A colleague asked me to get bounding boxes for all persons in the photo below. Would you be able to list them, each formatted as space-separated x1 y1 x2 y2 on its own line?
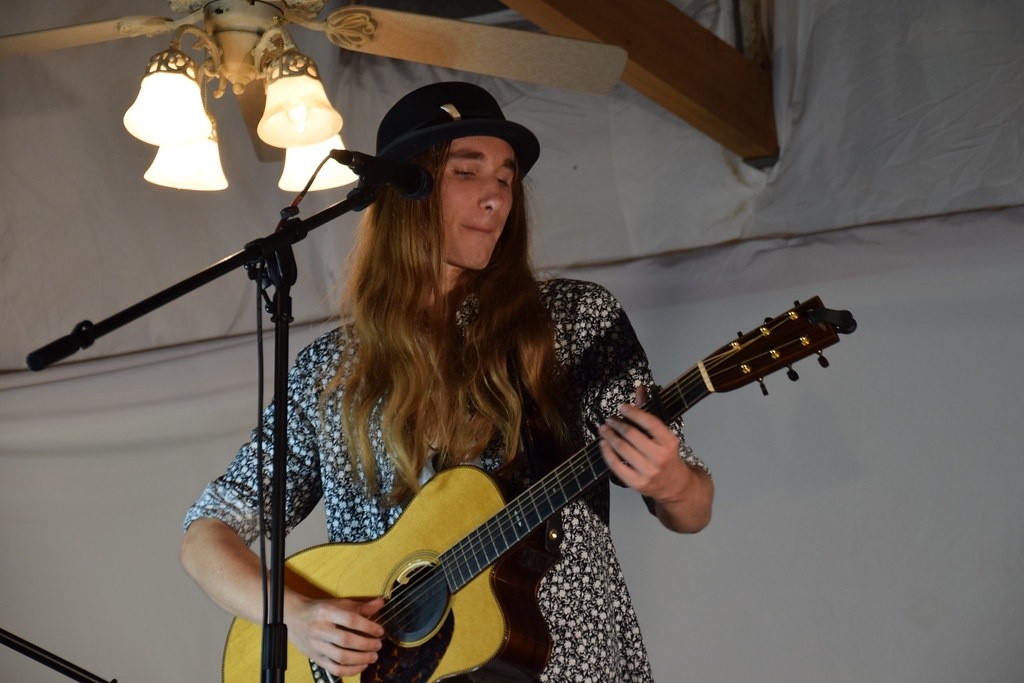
181 81 715 683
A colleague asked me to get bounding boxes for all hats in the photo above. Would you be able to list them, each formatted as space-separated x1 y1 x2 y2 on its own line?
376 82 540 179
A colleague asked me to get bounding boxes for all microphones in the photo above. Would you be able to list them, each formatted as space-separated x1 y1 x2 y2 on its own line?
330 150 434 200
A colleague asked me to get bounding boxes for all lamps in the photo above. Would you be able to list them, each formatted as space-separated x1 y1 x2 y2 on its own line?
123 23 359 193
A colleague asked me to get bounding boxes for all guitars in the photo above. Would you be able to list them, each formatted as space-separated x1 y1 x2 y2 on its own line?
219 294 860 683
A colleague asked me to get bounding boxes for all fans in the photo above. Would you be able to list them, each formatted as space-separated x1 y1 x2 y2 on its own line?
0 0 627 162
